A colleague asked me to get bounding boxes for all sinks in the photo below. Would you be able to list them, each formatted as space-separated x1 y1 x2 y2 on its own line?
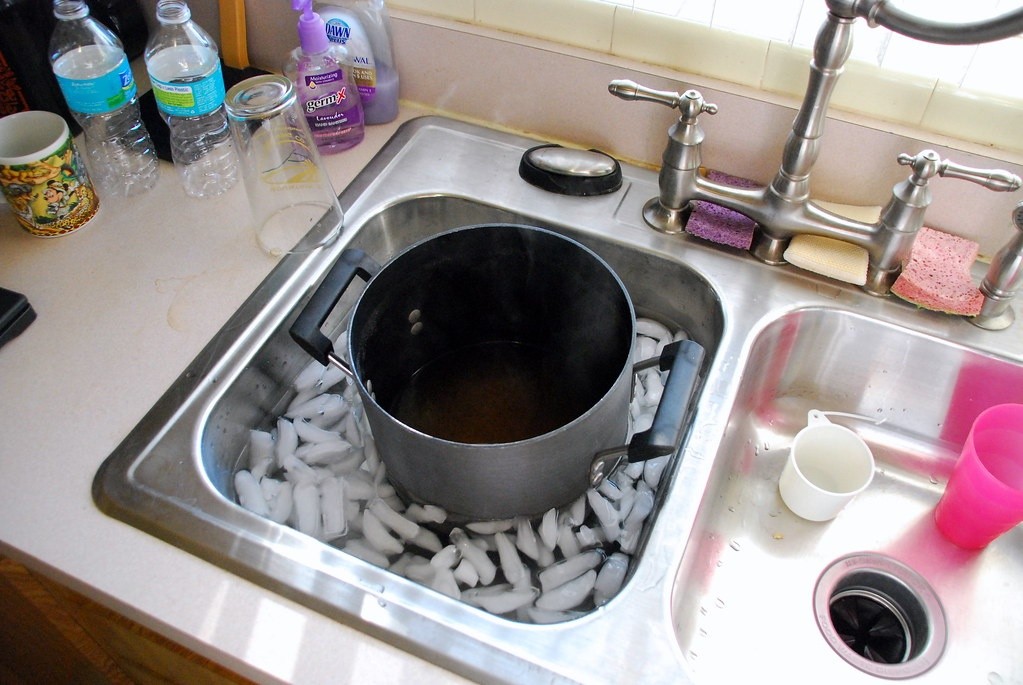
91 115 729 685
664 302 1023 685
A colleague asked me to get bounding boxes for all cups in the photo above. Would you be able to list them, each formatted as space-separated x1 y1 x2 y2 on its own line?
778 409 875 522
933 402 1022 551
224 74 344 254
0 110 101 239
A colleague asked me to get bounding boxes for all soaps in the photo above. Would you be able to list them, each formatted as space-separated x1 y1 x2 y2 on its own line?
528 147 617 177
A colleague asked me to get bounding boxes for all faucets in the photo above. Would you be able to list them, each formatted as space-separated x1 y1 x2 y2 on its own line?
604 0 1023 298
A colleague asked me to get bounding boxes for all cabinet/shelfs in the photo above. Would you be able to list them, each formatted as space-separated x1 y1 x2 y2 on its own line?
0 556 257 685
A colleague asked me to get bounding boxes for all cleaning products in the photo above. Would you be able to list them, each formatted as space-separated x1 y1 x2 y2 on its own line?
280 1 402 158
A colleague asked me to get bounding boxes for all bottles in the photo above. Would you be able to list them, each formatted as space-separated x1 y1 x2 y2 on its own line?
48 0 159 198
312 0 400 125
144 0 240 200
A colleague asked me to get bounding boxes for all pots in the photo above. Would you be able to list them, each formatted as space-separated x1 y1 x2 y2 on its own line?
287 222 706 534
